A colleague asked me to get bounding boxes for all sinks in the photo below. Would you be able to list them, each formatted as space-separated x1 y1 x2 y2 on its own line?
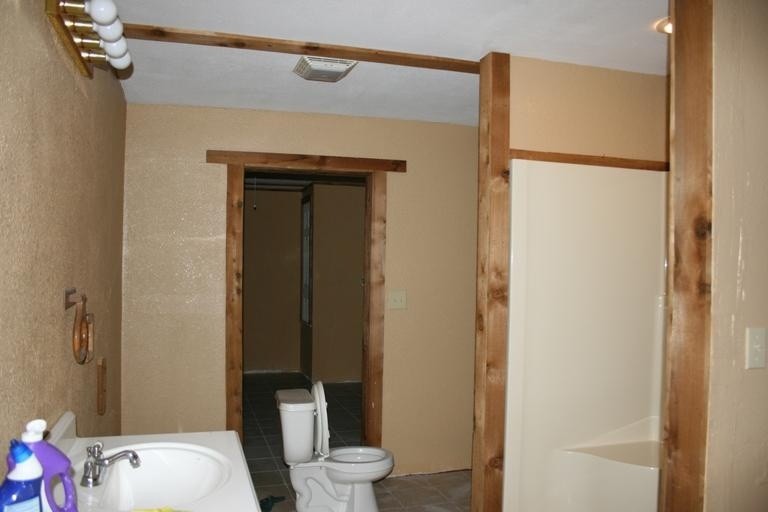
98 441 233 512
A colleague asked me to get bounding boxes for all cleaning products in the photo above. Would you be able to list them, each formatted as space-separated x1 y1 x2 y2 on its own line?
0 419 76 512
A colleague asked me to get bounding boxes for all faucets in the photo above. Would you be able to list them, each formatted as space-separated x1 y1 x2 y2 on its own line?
93 449 142 478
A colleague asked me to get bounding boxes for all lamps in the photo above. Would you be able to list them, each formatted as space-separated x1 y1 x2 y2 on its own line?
47 0 135 77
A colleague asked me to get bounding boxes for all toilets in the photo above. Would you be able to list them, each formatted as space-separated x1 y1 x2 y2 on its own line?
276 381 394 512
